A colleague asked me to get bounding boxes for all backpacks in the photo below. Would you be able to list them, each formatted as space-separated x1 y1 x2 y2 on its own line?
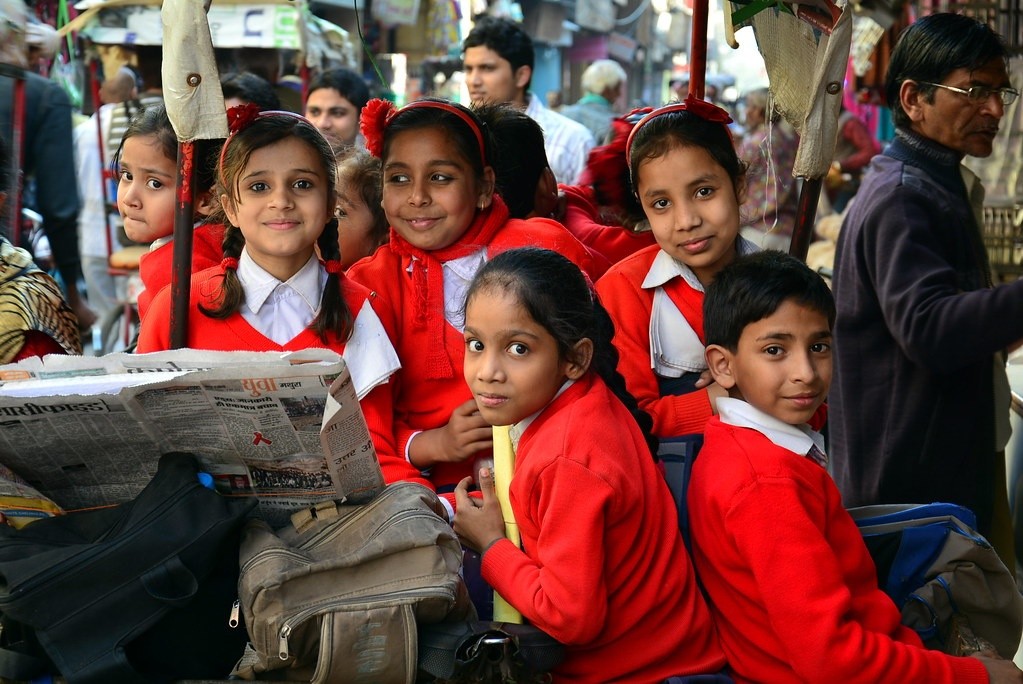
0 450 257 684
229 478 477 684
848 502 1023 659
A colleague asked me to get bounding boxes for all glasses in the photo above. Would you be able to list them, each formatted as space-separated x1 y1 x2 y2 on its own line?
920 79 1020 106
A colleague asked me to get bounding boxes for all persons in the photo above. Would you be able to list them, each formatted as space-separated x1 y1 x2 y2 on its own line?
673 74 873 253
562 106 654 265
0 139 83 363
109 108 231 317
688 251 1023 684
475 100 558 221
334 151 392 266
594 95 830 518
73 70 167 354
462 19 630 189
0 0 99 329
432 245 730 684
344 98 592 616
221 68 368 160
828 13 1023 572
138 111 434 490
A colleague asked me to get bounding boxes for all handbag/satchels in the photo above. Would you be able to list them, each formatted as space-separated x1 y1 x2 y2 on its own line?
416 619 559 684
